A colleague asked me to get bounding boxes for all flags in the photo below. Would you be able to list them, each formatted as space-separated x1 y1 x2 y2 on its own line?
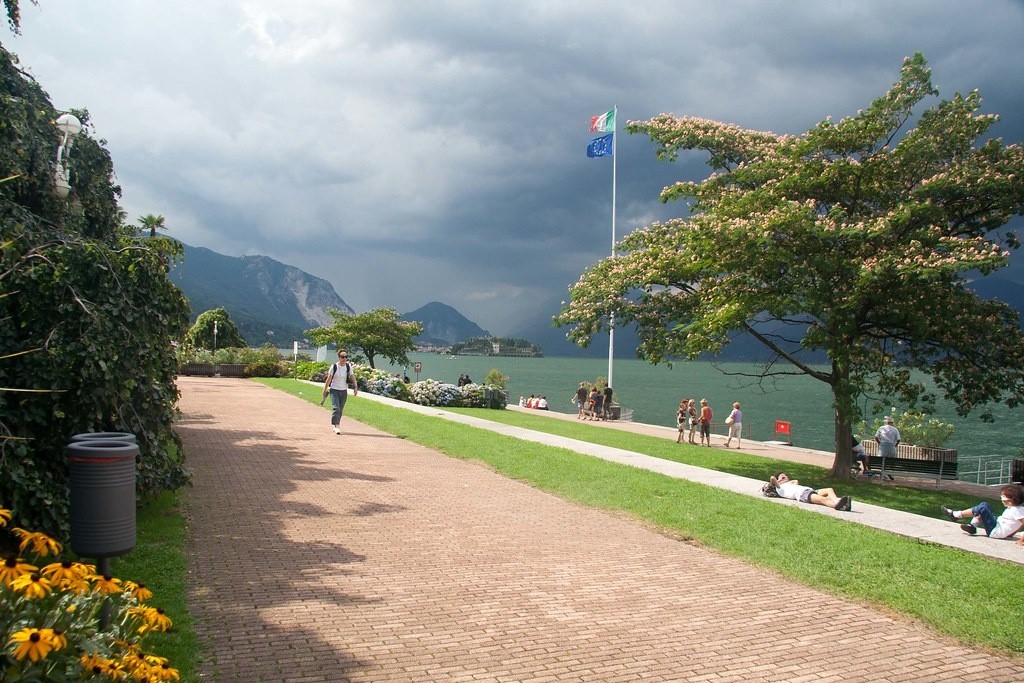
586 132 614 158
588 109 615 132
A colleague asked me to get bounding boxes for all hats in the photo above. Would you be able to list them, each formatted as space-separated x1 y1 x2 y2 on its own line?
886 418 894 422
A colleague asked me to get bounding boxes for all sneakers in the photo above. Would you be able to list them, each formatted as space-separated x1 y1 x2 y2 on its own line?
835 496 848 510
844 496 852 511
334 428 341 435
941 505 958 523
961 523 976 534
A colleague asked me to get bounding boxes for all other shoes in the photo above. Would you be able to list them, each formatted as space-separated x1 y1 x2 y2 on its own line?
888 475 895 480
677 440 740 448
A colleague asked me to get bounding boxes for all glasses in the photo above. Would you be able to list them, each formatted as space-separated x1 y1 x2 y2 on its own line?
338 355 347 358
777 476 789 482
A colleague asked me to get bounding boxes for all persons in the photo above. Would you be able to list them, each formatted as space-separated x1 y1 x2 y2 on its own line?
770 471 851 511
677 398 713 447
458 374 472 387
519 394 548 410
941 484 1024 546
323 349 358 435
405 376 410 383
724 402 743 449
573 382 613 421
851 434 873 476
874 417 901 481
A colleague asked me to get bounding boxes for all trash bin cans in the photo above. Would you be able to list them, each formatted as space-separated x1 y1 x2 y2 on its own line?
610 405 620 420
65 432 138 558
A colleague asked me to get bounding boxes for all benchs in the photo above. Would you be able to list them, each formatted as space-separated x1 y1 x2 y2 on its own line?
853 451 958 489
